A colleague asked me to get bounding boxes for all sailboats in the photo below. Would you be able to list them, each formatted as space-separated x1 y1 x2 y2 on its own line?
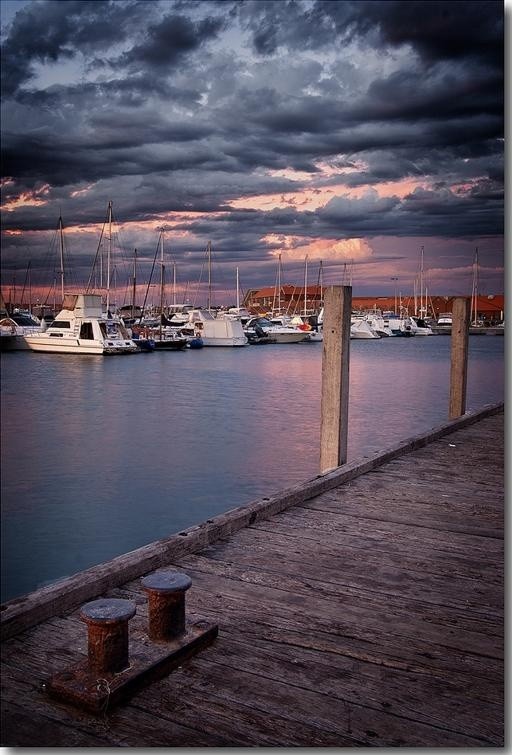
0 200 504 353
23 201 138 356
